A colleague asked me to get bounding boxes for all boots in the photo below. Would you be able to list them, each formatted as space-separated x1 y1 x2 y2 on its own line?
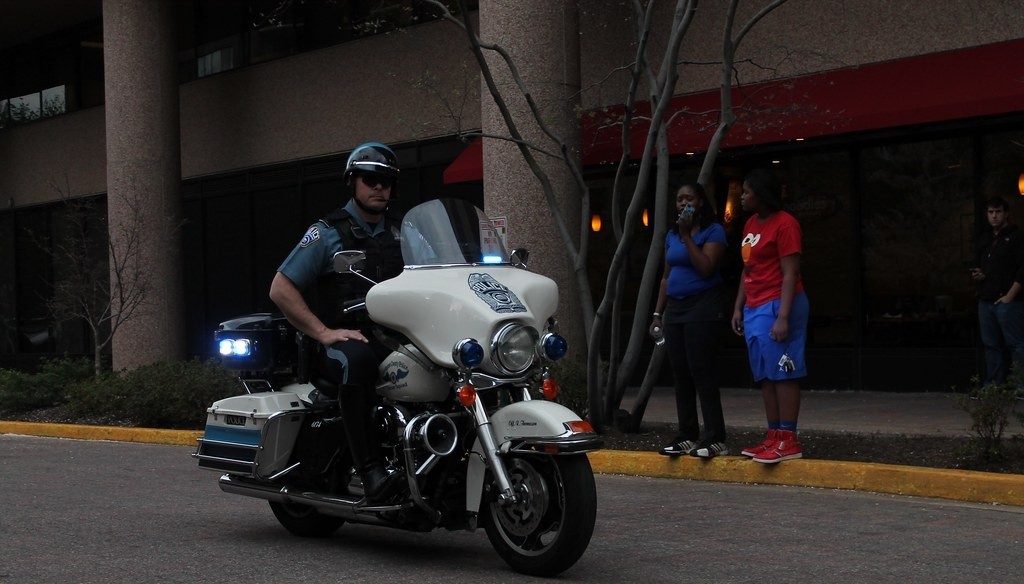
338 383 408 505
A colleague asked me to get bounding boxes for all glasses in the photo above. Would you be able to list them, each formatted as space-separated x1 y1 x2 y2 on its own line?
358 175 394 189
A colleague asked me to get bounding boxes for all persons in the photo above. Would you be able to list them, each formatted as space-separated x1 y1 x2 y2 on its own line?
728 171 810 464
269 144 437 506
646 182 732 457
970 197 1024 400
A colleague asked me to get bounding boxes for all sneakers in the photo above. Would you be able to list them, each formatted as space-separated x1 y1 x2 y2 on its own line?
657 440 701 455
741 429 803 462
689 440 729 458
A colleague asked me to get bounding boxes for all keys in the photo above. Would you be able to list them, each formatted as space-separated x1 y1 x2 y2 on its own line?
779 355 795 373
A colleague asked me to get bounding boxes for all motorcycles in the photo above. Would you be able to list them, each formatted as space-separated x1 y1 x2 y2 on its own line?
193 200 596 578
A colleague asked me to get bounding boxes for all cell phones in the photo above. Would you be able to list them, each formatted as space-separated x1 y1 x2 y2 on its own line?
969 269 977 273
682 204 696 215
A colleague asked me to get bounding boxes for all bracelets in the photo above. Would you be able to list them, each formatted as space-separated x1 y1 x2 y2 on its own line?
651 313 660 317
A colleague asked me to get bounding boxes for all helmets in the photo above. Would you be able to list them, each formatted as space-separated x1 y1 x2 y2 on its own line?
343 142 401 177
